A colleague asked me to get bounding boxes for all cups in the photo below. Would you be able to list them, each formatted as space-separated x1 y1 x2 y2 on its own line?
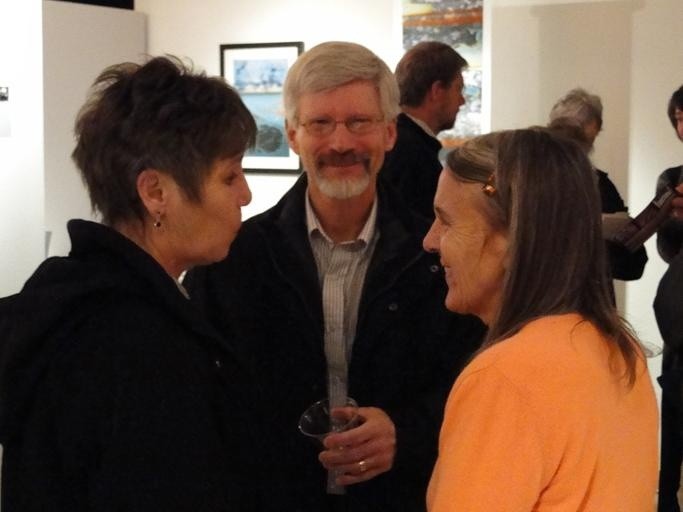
299 397 359 450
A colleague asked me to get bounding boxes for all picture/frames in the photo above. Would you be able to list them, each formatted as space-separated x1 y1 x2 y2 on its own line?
218 42 306 175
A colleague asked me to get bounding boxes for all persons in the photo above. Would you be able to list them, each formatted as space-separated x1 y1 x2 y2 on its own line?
549 86 650 282
653 83 682 511
424 125 657 509
181 43 487 509
373 41 470 236
2 55 262 509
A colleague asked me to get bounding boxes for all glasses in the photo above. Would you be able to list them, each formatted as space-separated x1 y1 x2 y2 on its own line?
300 115 384 136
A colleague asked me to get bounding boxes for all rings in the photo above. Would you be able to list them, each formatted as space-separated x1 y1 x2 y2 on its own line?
359 461 366 472
